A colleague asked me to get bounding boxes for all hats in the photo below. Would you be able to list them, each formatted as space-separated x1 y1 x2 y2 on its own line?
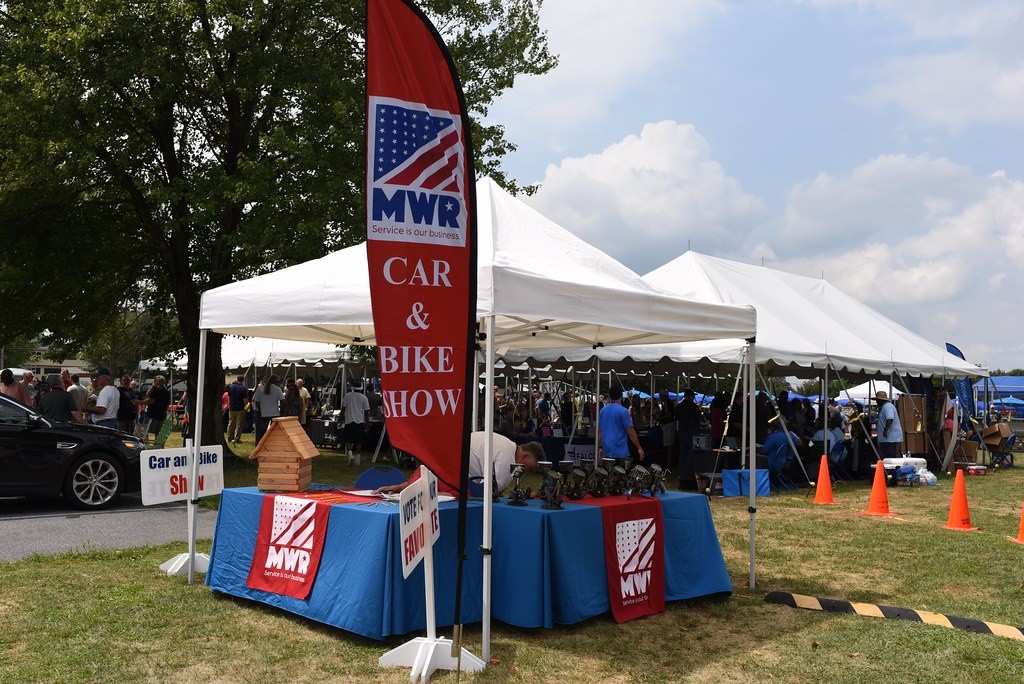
684 389 698 396
351 377 364 390
91 367 111 378
870 391 891 402
155 375 165 382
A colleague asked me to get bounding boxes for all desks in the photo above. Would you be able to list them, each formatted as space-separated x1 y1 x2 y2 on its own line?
308 418 389 450
207 480 486 640
468 491 734 630
516 430 664 469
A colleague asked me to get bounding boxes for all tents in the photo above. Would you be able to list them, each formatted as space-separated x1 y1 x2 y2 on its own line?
137 172 992 661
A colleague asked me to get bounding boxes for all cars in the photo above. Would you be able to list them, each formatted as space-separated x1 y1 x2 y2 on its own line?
166 380 188 413
46 374 61 382
139 382 152 421
0 391 148 511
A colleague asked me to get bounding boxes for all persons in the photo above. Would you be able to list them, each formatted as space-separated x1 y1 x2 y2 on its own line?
0 366 904 481
371 462 471 500
982 403 1003 431
469 429 546 494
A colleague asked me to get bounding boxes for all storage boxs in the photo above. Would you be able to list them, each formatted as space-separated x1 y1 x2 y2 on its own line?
944 420 1013 475
871 454 926 486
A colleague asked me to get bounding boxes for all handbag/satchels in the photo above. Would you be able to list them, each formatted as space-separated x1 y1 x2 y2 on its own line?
647 420 663 443
917 466 938 486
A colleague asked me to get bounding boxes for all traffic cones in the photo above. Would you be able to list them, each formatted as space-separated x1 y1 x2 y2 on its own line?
939 469 979 530
1005 499 1024 545
860 460 899 517
807 455 845 506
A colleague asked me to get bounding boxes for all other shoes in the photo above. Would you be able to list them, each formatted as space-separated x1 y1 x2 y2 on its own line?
236 440 242 443
348 456 355 466
354 460 360 466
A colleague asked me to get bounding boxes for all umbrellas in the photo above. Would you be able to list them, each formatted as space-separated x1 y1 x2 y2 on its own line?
622 387 1024 414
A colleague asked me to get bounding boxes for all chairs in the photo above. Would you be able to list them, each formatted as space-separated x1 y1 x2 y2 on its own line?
143 419 174 449
990 434 1016 472
137 419 153 439
765 444 800 493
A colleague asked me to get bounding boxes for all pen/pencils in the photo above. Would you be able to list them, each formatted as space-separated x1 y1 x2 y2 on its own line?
304 494 332 498
356 502 369 506
320 496 357 504
379 489 393 494
282 493 307 496
381 502 389 507
376 502 379 508
368 502 376 506
390 501 397 506
383 499 400 500
